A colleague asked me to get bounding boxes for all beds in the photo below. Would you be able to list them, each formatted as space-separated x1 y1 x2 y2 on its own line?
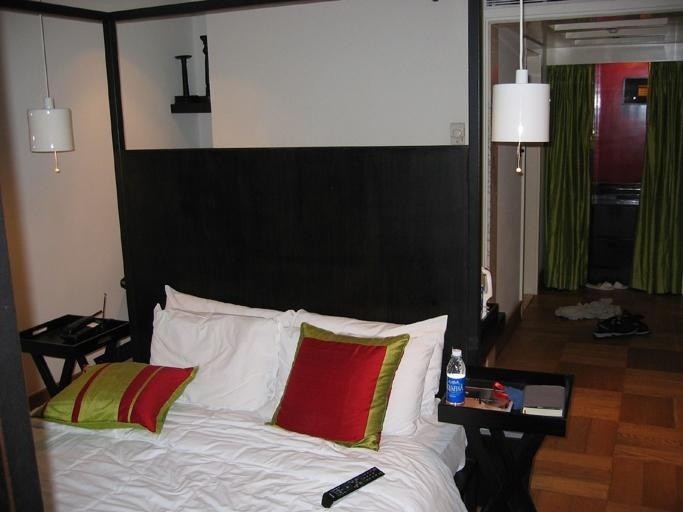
0 146 483 512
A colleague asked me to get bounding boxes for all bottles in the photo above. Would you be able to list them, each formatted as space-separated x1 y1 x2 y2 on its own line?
444 349 467 405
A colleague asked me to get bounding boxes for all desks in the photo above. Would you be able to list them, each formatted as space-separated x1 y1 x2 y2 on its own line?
436 365 575 511
19 311 129 401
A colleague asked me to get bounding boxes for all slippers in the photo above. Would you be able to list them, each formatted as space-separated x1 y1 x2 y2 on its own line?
585 281 629 293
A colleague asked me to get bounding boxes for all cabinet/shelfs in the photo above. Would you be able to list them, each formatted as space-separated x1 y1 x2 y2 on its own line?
588 197 640 272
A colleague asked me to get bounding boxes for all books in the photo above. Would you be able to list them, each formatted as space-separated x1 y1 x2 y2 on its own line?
522 385 568 417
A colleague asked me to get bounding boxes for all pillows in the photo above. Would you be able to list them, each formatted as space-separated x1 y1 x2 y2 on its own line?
296 308 450 425
162 283 296 334
267 321 410 453
148 300 289 420
264 316 438 436
29 355 199 436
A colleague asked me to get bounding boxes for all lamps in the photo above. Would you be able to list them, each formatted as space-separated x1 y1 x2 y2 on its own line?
27 15 75 175
489 0 557 173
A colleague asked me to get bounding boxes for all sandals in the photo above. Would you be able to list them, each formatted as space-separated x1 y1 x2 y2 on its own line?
621 309 649 336
592 315 638 338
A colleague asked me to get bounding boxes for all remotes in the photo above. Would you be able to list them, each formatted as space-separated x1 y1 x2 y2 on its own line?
321 466 384 509
